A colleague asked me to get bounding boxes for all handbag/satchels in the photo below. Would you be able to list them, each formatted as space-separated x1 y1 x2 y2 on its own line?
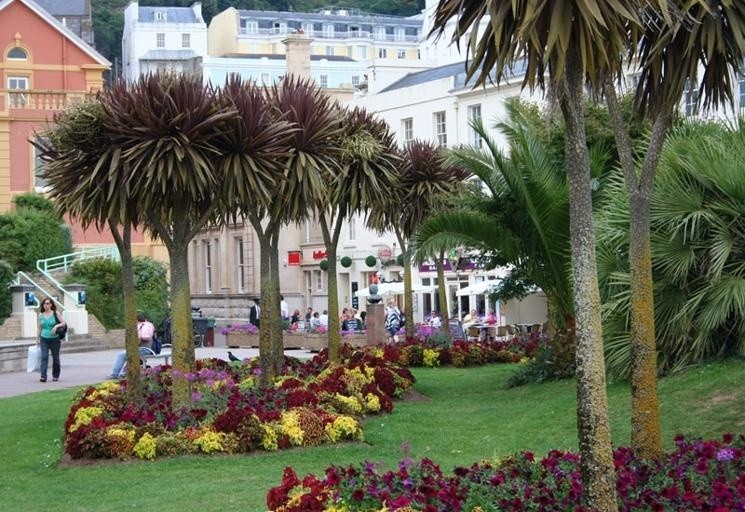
53 309 68 339
153 331 163 354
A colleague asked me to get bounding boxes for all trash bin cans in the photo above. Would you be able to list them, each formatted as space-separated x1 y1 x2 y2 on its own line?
207 327 214 346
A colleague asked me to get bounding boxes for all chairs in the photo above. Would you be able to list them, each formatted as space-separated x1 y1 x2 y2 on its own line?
464 322 540 342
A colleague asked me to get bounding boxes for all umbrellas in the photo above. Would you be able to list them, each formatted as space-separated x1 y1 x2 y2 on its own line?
455 278 543 308
356 282 439 296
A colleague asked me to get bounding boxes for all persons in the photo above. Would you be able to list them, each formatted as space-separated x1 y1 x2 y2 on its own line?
429 309 440 329
482 307 497 342
463 308 479 343
249 294 405 336
36 298 65 381
108 314 157 379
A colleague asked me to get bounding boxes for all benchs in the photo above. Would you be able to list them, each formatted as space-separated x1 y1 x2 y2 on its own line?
136 343 172 369
